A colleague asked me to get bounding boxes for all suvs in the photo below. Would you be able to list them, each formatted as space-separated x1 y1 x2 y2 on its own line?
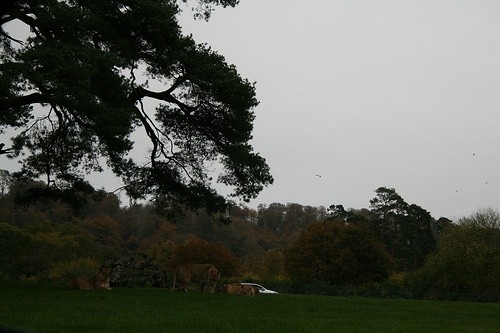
240 282 280 295
